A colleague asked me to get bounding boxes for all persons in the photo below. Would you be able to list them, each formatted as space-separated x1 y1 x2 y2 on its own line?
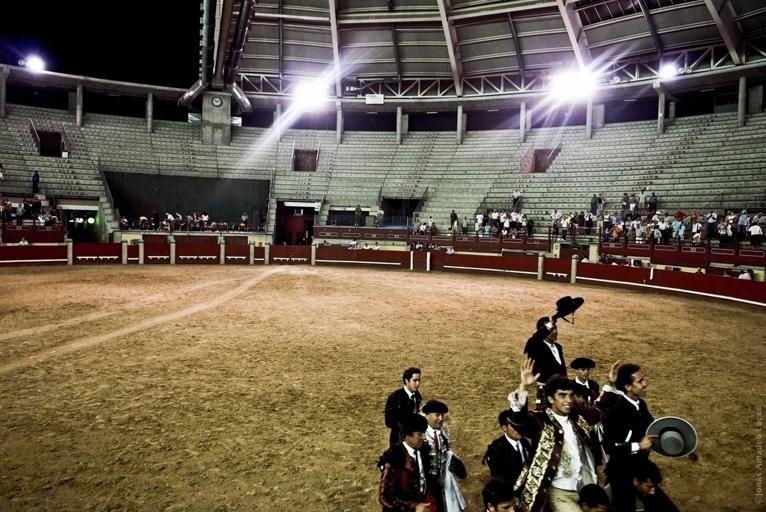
121 212 248 235
2 196 60 228
482 316 679 510
0 163 5 181
355 205 361 226
378 366 467 509
411 170 765 280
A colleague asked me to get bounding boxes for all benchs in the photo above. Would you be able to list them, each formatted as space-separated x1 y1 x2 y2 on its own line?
0 103 766 253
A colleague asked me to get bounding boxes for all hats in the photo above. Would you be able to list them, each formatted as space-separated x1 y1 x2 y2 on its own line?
570 358 595 368
645 417 698 458
551 296 584 320
422 400 448 413
397 414 427 430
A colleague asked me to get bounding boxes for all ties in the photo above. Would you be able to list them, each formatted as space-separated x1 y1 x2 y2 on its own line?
434 431 439 455
516 444 522 463
412 394 418 414
